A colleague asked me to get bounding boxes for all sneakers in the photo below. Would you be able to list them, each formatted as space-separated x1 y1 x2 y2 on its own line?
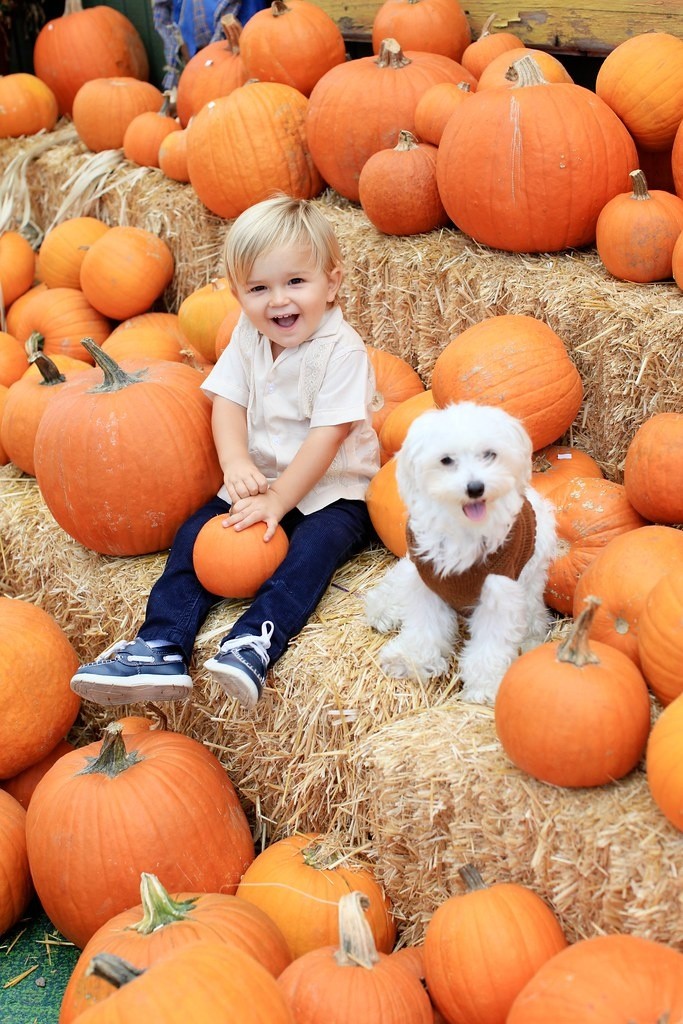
69 636 193 706
202 620 274 710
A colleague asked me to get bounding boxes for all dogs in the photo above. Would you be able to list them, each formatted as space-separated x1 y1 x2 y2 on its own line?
363 400 561 705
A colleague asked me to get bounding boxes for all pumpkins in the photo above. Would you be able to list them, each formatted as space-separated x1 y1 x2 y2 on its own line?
0 0 683 1024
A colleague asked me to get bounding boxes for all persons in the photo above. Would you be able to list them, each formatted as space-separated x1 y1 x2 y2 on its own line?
70 188 381 709
